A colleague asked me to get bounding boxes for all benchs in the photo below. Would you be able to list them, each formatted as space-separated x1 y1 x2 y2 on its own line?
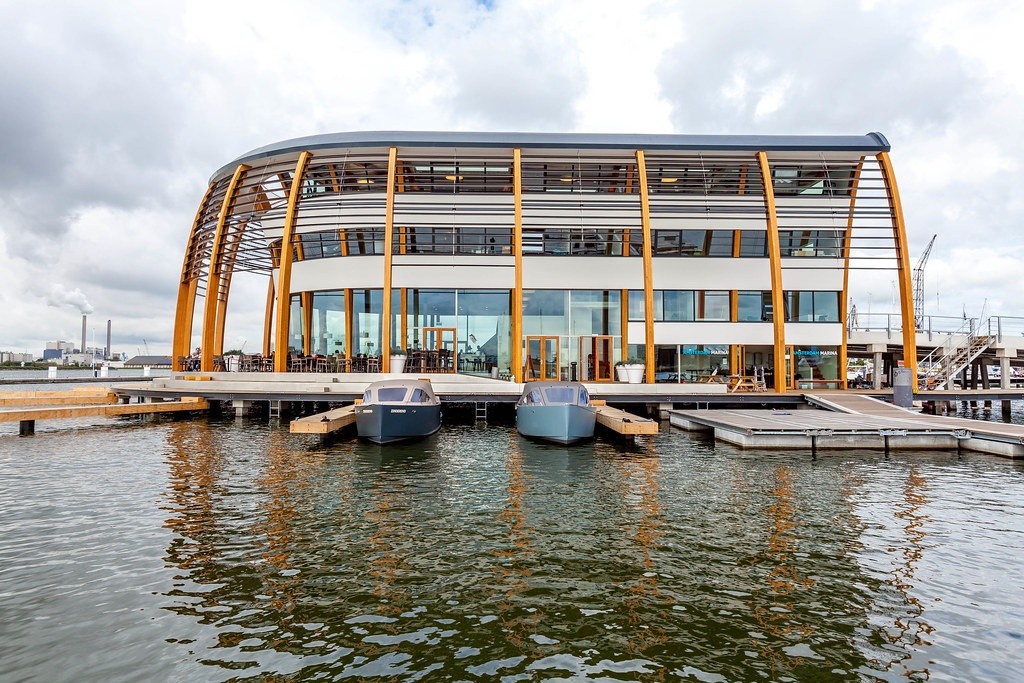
692 381 763 392
795 379 844 390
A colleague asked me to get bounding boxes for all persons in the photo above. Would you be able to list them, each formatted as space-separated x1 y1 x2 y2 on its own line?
287 351 292 367
192 348 200 372
332 350 340 362
458 349 464 361
267 351 275 363
477 345 485 354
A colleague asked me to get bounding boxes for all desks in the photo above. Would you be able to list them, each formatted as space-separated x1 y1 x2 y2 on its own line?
726 375 763 393
686 368 712 382
697 374 731 392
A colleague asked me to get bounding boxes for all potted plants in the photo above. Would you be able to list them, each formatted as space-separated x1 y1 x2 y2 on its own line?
221 349 244 371
622 355 645 383
390 348 408 373
614 361 628 382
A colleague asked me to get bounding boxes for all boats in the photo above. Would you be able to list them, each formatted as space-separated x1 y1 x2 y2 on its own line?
518 381 599 448
355 380 443 446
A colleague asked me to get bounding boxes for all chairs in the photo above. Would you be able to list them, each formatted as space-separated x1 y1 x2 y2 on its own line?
177 348 497 373
